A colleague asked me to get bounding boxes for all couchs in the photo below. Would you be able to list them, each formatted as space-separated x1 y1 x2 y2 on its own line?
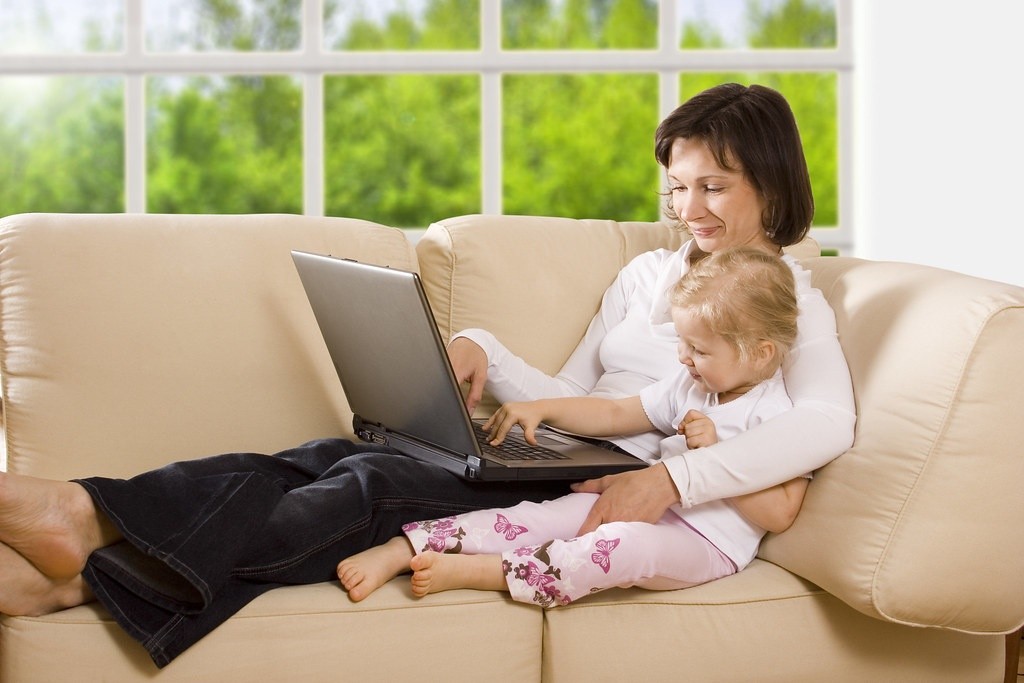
0 211 1024 683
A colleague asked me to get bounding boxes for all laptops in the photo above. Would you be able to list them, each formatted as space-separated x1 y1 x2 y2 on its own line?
289 250 652 481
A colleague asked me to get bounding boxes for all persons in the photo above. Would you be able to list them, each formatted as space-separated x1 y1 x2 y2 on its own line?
0 81 859 670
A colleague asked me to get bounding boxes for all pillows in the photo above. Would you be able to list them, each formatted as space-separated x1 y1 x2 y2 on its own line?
757 255 1024 636
415 212 823 419
0 211 424 479
